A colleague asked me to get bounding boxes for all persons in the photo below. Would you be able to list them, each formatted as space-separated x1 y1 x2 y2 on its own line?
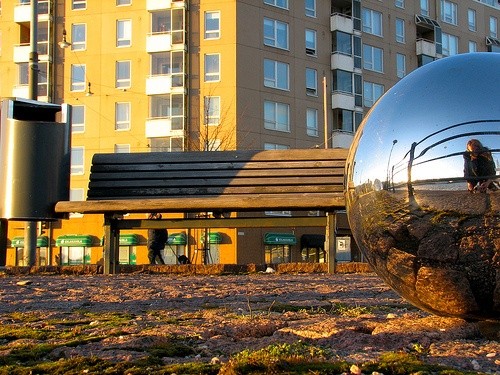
146 212 170 265
462 138 499 194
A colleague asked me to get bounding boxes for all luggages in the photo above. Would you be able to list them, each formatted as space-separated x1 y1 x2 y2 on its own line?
166 242 191 264
